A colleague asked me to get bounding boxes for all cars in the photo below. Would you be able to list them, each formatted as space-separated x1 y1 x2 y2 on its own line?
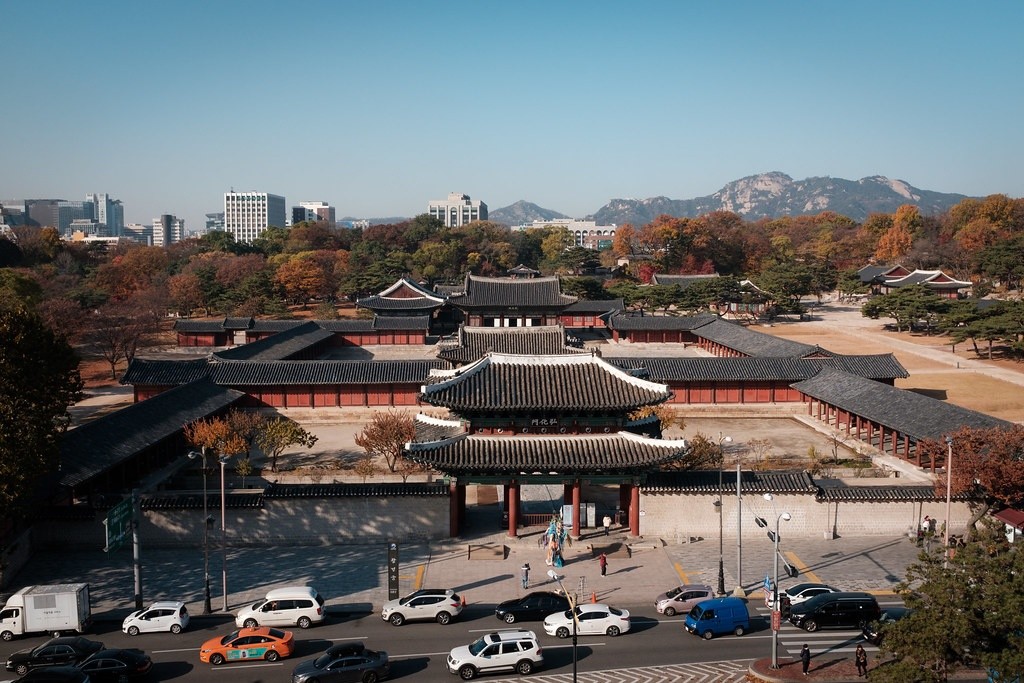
543 603 632 638
766 582 841 612
494 591 571 624
121 601 190 636
654 584 713 616
200 627 295 665
73 647 153 683
235 586 327 629
5 635 105 675
0 666 90 683
864 605 912 649
291 641 389 683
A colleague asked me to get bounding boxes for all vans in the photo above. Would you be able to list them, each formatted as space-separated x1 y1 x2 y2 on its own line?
683 597 751 640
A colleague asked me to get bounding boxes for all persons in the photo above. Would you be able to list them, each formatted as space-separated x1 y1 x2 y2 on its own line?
855 645 868 679
271 601 276 611
949 534 967 548
800 644 810 675
603 514 611 536
537 514 572 568
521 563 530 589
923 516 931 531
600 552 608 576
941 520 946 538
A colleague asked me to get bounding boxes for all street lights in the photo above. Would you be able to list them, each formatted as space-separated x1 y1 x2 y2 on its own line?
716 430 733 596
548 570 582 683
768 512 793 671
942 436 952 576
187 445 210 616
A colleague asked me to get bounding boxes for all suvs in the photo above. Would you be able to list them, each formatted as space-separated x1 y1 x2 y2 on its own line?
381 587 463 625
786 587 877 634
445 630 544 680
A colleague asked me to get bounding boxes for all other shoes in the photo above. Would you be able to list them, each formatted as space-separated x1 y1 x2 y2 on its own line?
803 672 806 675
525 587 528 589
859 674 862 677
601 574 605 577
865 676 868 679
806 672 810 675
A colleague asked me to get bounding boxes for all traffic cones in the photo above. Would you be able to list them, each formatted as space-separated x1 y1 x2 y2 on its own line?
592 592 598 604
461 595 468 608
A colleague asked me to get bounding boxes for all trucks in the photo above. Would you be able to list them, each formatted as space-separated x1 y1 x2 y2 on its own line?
0 582 93 641
